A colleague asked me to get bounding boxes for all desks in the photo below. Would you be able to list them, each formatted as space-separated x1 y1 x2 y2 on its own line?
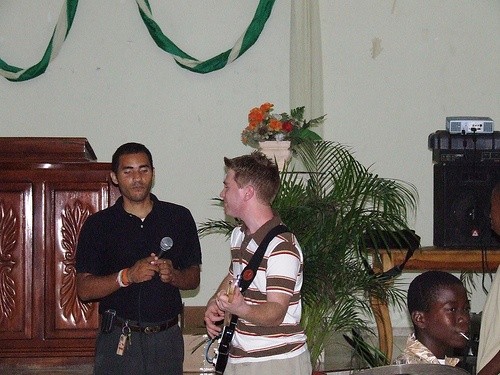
368 247 500 366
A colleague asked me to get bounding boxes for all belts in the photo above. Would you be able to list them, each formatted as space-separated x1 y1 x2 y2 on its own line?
104 318 178 335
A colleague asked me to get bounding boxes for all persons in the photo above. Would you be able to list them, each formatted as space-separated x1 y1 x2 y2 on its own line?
75 143 202 375
478 182 500 375
393 272 472 366
204 154 312 375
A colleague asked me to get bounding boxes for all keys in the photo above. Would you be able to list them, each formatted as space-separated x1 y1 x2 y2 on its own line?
125 334 132 351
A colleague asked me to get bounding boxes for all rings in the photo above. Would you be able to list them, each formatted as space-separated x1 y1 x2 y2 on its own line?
163 259 167 264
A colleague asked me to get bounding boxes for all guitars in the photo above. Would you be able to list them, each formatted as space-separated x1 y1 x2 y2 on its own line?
212 274 241 375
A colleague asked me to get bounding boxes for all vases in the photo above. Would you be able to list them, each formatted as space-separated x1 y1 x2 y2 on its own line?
258 140 294 172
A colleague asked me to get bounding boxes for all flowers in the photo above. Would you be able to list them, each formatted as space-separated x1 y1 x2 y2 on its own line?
239 101 326 148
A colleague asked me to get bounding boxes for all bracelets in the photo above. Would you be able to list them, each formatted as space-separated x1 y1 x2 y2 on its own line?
118 268 130 288
